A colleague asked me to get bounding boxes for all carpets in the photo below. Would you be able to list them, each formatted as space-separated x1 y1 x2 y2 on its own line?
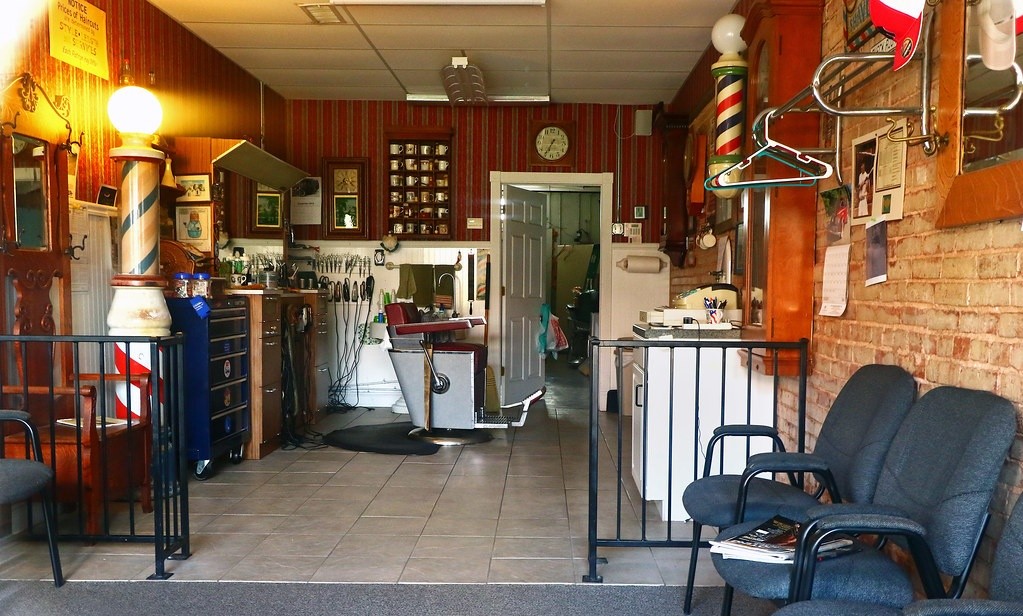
0 581 781 616
322 420 438 456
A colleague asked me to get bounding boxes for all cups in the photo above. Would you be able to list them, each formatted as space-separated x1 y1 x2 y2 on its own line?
706 309 724 324
752 309 762 323
695 229 716 249
299 279 305 289
230 273 246 286
309 278 314 288
390 144 448 233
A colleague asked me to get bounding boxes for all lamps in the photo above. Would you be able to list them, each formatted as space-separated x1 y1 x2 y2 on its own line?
406 94 551 102
107 85 165 288
439 57 487 105
707 13 748 202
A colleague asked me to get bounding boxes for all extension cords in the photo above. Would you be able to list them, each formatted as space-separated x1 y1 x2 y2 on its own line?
683 323 733 330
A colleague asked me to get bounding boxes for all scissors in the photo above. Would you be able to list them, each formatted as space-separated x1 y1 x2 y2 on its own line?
317 252 371 277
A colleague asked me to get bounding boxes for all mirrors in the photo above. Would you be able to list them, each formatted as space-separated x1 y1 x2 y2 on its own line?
933 0 1023 229
210 164 288 289
0 70 73 418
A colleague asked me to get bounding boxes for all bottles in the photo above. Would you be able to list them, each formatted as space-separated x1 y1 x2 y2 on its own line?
218 252 249 277
374 288 396 324
259 266 277 288
192 273 210 297
173 272 191 297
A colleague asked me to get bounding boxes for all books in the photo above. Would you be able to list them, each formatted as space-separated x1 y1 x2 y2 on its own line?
709 514 856 566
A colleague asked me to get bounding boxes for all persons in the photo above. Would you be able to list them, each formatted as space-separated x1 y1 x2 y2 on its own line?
858 162 870 216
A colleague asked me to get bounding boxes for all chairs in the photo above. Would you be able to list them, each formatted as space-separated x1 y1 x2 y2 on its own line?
681 362 1023 616
386 303 547 446
0 265 154 548
0 409 64 587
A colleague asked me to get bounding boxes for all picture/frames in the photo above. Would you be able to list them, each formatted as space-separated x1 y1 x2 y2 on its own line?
245 180 286 240
320 155 370 238
175 205 211 253
171 175 212 204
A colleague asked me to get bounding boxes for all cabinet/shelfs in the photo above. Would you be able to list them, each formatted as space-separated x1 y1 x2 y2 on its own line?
385 127 454 239
164 138 328 478
555 244 601 352
631 0 822 523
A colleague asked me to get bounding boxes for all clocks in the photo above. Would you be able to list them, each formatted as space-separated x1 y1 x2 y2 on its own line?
529 119 576 167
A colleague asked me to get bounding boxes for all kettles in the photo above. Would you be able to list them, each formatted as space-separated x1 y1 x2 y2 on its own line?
300 304 312 327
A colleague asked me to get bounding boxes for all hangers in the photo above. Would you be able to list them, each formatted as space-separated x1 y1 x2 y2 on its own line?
704 111 834 190
